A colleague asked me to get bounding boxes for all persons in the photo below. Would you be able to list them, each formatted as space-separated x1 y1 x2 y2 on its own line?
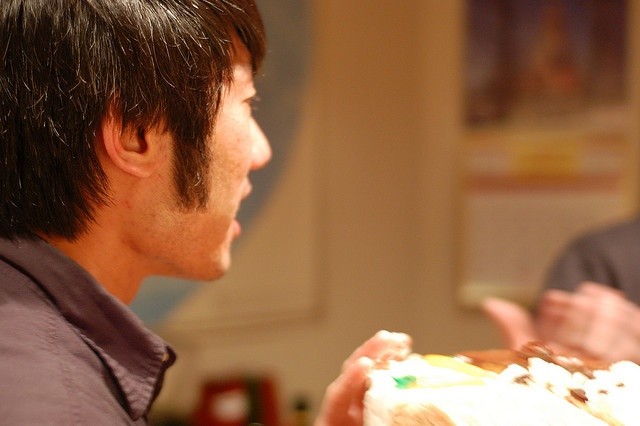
0 0 413 426
480 213 640 368
